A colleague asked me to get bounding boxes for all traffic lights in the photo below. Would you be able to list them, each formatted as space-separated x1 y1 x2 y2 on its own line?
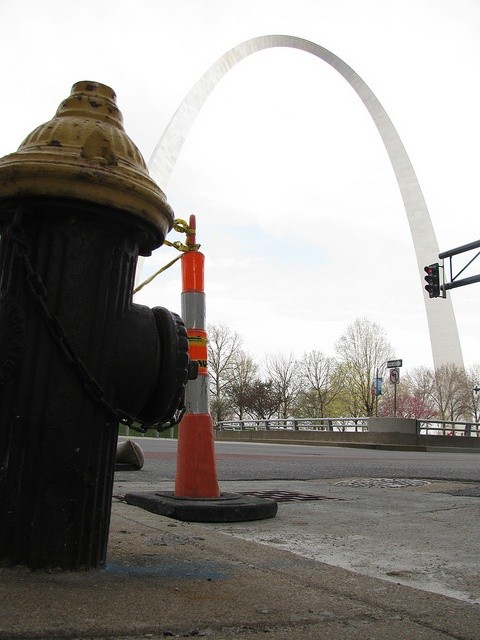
424 263 440 298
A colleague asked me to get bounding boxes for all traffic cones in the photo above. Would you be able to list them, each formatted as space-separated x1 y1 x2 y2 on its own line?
125 213 279 521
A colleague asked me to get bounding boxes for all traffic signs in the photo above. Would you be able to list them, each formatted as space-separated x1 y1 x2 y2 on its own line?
387 359 402 368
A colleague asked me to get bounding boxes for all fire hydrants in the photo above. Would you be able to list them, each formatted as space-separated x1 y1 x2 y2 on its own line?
0 81 197 572
